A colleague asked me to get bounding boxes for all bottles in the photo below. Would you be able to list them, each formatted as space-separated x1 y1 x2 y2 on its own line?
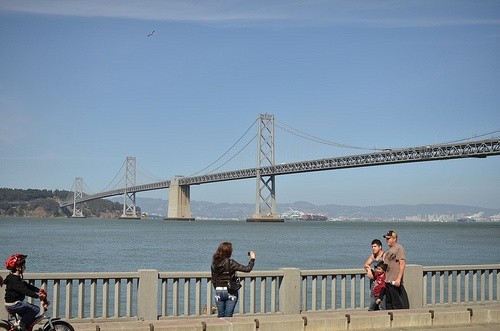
378 301 385 310
389 280 396 286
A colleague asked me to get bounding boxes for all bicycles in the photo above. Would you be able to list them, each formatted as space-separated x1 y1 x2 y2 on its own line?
0 288 77 331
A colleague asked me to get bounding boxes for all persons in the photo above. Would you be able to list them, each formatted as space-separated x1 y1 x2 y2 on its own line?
364 239 386 310
365 261 388 311
3 254 46 331
211 242 256 317
383 231 409 309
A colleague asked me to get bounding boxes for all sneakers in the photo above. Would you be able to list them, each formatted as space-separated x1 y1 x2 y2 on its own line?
13 320 26 331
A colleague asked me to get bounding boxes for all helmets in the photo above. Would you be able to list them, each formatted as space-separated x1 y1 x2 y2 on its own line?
4 254 27 269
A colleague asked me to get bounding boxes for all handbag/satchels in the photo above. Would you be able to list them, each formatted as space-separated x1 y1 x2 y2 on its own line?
227 259 242 293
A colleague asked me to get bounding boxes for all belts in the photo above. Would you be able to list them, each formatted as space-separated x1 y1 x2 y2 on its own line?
216 286 228 291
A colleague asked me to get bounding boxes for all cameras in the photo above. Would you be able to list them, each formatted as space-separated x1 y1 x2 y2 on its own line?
248 252 250 256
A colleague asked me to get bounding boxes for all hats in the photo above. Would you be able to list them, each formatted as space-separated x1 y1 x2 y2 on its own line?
383 231 397 238
